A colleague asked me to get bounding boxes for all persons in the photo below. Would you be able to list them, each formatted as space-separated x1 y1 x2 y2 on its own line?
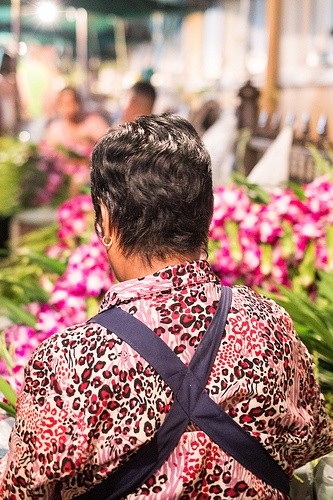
0 113 333 499
1 54 163 155
